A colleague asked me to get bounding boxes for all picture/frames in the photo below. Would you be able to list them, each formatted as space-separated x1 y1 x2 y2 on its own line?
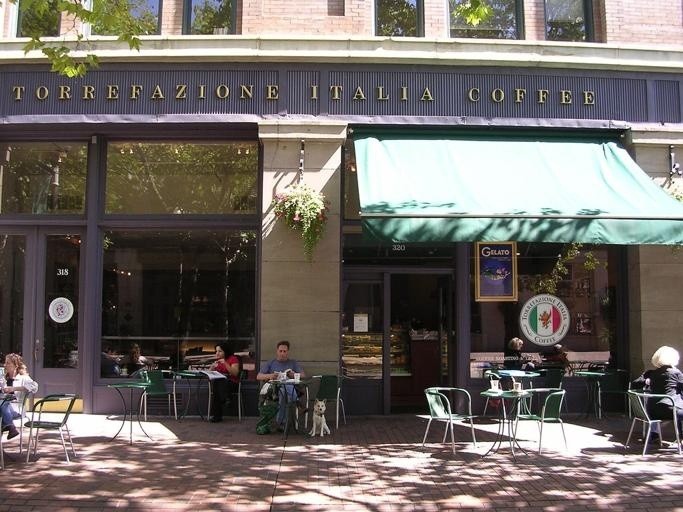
474 240 517 302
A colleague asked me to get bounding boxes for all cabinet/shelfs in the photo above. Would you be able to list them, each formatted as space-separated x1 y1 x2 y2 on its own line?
340 332 412 377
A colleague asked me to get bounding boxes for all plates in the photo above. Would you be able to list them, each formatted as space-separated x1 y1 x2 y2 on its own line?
488 389 499 393
511 390 524 393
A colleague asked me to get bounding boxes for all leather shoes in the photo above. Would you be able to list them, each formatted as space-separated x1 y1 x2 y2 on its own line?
7 430 19 439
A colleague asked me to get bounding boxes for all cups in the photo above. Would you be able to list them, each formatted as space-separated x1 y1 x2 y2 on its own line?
294 373 300 382
119 368 127 374
513 382 521 390
490 380 499 390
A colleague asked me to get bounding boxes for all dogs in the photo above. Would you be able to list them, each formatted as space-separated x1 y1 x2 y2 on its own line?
308 398 330 437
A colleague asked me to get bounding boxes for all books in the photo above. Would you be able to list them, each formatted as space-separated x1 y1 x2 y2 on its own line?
185 370 228 380
268 368 291 383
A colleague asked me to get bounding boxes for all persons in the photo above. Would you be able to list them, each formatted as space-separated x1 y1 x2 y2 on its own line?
504 337 530 369
209 342 240 423
0 353 39 439
257 341 305 432
101 342 155 377
632 345 683 451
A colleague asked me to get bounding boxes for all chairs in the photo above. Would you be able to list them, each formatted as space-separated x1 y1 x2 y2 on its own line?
421 358 683 462
0 392 81 468
105 357 248 443
255 370 348 442
